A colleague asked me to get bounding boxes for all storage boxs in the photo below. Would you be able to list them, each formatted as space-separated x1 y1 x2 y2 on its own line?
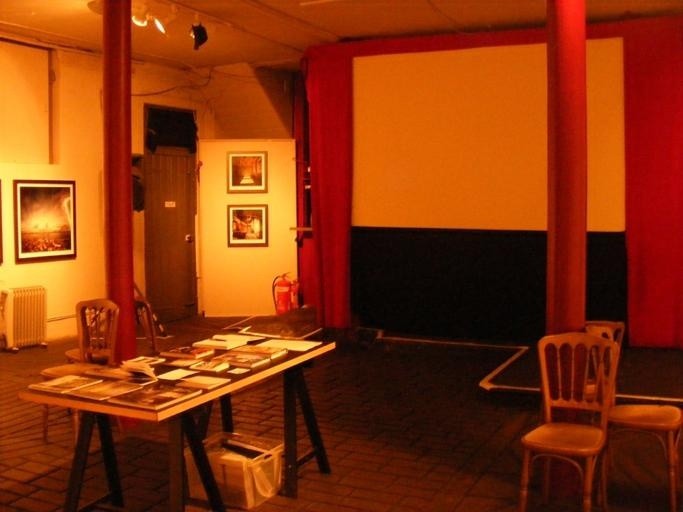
182 429 285 509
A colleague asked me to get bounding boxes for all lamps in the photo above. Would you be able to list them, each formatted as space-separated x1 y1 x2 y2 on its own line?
134 1 210 51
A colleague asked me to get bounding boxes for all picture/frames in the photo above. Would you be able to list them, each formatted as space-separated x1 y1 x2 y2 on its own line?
226 150 269 194
226 204 270 247
13 179 78 265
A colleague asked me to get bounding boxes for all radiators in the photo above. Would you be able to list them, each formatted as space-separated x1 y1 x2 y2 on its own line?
2 286 46 350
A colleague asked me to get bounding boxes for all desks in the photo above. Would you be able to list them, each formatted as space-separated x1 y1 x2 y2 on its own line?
19 337 338 512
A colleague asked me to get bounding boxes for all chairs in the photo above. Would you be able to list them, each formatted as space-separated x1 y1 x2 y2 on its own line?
40 300 123 450
518 318 683 510
138 298 163 353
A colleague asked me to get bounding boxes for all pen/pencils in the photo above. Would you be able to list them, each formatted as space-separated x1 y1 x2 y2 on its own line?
238 325 252 333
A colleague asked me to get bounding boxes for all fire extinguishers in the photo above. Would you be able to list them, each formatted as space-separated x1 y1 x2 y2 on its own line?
272 274 298 315
